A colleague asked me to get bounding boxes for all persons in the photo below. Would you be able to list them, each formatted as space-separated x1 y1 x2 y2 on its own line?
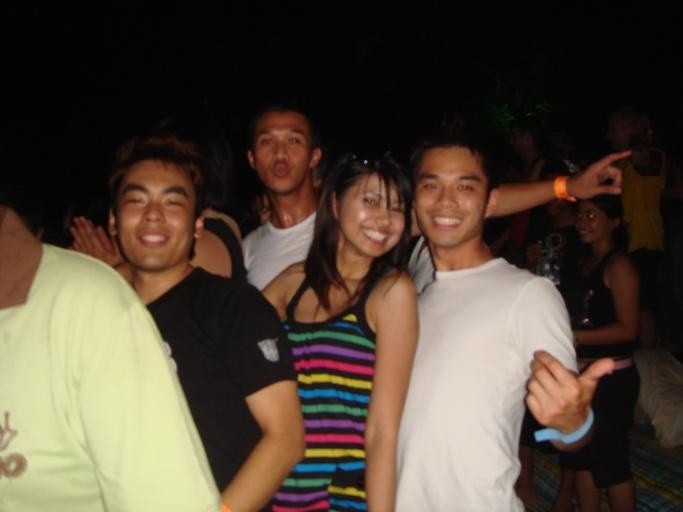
0 79 682 512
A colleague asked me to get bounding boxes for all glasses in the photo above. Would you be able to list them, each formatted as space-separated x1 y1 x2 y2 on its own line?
336 141 417 179
